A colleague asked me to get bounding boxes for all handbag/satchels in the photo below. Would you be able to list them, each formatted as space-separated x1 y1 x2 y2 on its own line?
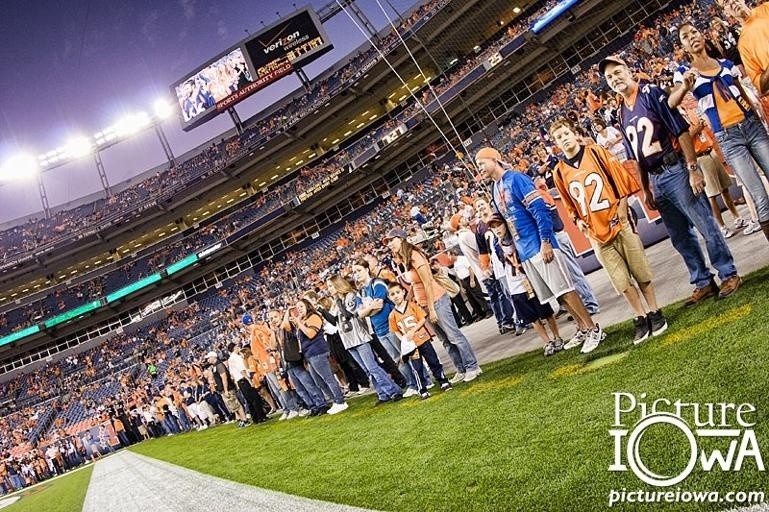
431 269 460 298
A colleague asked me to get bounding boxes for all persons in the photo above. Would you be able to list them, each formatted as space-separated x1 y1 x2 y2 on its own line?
0 145 205 285
242 316 299 421
268 310 330 416
598 56 741 308
384 146 475 241
669 87 751 239
385 282 453 399
206 275 243 349
447 248 493 322
475 82 559 189
228 343 273 424
206 351 251 427
176 48 252 121
668 23 769 240
563 14 668 121
351 260 418 397
549 120 667 345
315 197 384 276
451 214 516 334
205 90 315 249
475 147 601 353
289 299 348 414
244 248 315 321
668 0 742 66
315 33 385 182
716 0 769 94
488 213 564 355
316 275 402 405
504 0 563 49
592 119 627 162
430 259 474 328
385 0 504 132
383 229 482 383
0 285 206 498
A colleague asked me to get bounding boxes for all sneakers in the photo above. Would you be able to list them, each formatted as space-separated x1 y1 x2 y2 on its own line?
279 411 289 421
403 388 419 398
327 403 336 414
239 420 252 427
329 401 349 415
543 341 555 357
553 337 564 352
440 383 453 391
742 222 761 235
556 310 568 319
267 410 276 416
276 409 284 413
258 416 273 424
306 408 320 419
580 322 607 354
486 310 492 321
393 394 403 402
475 313 484 322
299 409 311 417
287 410 298 420
502 322 516 334
567 304 599 320
466 318 474 326
462 366 482 383
426 383 435 389
498 324 511 335
735 217 750 228
343 391 357 400
632 315 650 345
421 392 431 400
318 406 330 416
685 280 720 308
721 227 736 238
355 388 372 395
198 425 203 432
202 425 208 430
515 326 528 336
562 328 588 351
375 398 392 406
718 274 742 298
646 309 668 336
449 372 465 384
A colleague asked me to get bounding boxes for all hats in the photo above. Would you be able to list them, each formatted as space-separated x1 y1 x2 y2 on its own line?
205 351 217 358
243 316 254 324
599 55 626 75
486 213 504 227
450 214 463 230
476 147 510 168
383 227 406 245
456 202 463 206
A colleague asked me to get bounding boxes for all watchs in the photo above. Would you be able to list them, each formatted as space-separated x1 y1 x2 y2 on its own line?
541 238 552 244
573 216 579 226
687 163 698 171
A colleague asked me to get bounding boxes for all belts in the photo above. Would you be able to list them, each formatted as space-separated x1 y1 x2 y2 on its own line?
698 147 712 157
650 151 683 175
